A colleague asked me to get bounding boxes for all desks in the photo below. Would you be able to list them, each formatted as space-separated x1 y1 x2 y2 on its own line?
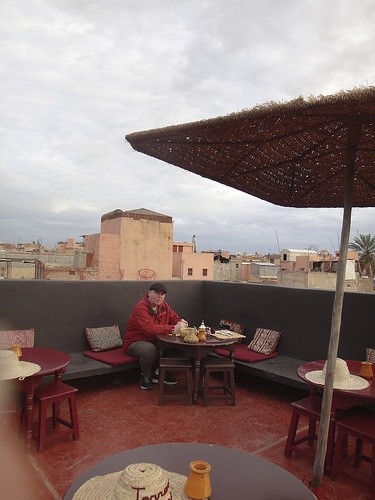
156 331 234 404
3 346 72 451
60 442 318 500
297 358 375 400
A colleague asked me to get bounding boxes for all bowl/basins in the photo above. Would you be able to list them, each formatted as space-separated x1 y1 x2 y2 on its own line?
180 328 197 338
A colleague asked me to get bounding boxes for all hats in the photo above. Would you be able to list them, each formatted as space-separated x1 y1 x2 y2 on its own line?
149 283 168 294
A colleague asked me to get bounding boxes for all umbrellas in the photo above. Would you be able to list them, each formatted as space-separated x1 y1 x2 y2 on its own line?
126 86 375 492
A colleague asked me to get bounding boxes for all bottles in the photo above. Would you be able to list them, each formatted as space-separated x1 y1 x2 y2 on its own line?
360 361 373 379
184 329 199 343
199 323 206 330
183 460 212 500
198 330 206 341
11 344 22 357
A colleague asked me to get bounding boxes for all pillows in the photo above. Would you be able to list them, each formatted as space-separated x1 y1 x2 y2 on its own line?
85 326 123 353
247 327 281 355
220 319 244 342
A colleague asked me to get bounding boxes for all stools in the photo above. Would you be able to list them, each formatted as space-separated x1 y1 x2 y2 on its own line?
283 396 375 486
157 359 194 405
18 380 79 454
199 357 235 406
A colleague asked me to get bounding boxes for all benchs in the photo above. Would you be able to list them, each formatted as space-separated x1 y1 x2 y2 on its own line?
0 277 375 392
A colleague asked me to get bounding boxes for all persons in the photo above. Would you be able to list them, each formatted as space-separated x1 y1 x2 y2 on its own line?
123 283 188 390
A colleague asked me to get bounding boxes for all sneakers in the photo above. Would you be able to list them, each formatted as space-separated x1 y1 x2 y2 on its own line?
139 372 152 390
152 372 178 385
